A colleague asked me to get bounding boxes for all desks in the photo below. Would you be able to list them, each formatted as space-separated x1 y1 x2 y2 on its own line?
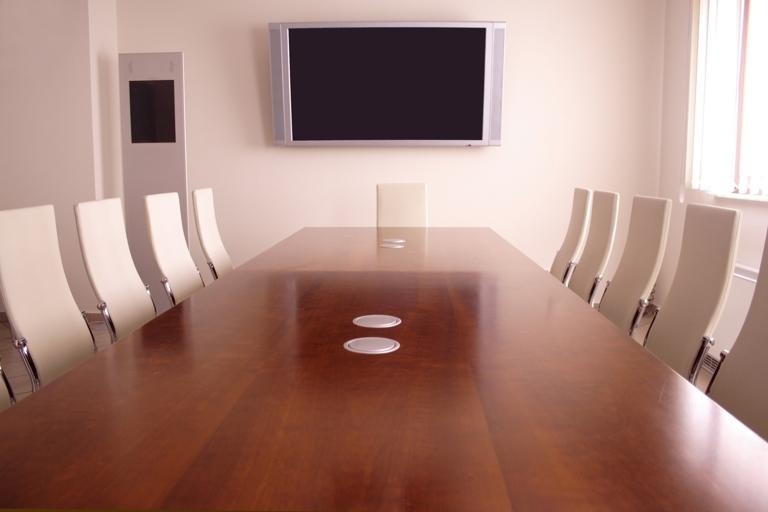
0 228 767 512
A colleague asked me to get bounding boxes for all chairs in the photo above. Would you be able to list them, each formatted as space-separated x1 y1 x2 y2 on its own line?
142 190 208 311
642 202 744 384
71 196 156 343
567 187 624 305
704 223 768 443
192 187 236 284
548 185 592 284
376 182 428 229
0 203 98 395
595 194 674 335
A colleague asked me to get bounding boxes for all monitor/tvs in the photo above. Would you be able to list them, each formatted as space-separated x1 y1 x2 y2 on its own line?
270 21 505 148
